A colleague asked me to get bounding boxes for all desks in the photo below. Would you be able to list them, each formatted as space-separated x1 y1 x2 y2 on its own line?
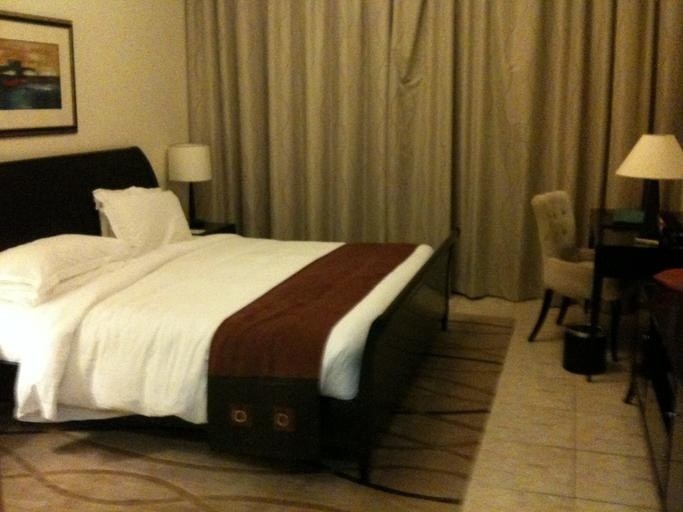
581 206 682 403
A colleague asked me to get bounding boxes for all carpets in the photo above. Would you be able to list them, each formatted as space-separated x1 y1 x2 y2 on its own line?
0 310 517 512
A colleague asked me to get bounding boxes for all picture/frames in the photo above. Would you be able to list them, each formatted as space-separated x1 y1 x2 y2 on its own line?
0 10 78 139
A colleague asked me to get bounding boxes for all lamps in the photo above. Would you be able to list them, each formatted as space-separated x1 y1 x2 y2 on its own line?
167 143 211 229
615 134 683 245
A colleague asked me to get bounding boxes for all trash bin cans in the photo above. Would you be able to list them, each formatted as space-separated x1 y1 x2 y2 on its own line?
562 323 610 375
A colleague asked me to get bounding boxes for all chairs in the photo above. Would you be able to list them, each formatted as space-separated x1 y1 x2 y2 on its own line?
527 190 622 361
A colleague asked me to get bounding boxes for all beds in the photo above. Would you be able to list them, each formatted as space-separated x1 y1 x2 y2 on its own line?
0 145 459 482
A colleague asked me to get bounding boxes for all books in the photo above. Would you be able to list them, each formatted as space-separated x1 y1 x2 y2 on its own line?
612 207 663 229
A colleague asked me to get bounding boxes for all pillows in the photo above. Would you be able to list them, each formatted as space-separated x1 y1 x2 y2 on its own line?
1 186 194 307
